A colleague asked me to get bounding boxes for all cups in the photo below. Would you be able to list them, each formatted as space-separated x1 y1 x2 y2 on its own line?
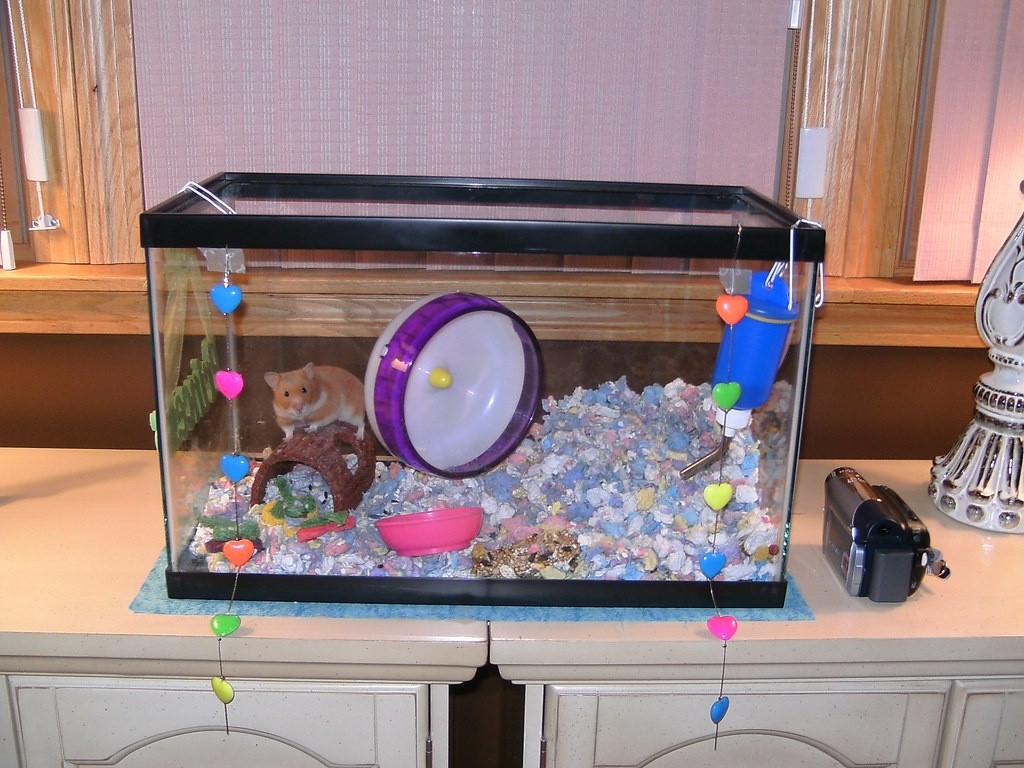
710 272 798 409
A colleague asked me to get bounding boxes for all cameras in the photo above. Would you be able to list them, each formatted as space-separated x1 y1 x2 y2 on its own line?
823 467 950 602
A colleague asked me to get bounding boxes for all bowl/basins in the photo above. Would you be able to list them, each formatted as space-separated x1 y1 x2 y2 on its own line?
374 507 484 555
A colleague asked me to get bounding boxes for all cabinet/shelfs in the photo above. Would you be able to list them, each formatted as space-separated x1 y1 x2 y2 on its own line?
0 452 487 768
487 459 1024 768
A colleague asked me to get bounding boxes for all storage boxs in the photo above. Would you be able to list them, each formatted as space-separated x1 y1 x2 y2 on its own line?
140 173 827 608
